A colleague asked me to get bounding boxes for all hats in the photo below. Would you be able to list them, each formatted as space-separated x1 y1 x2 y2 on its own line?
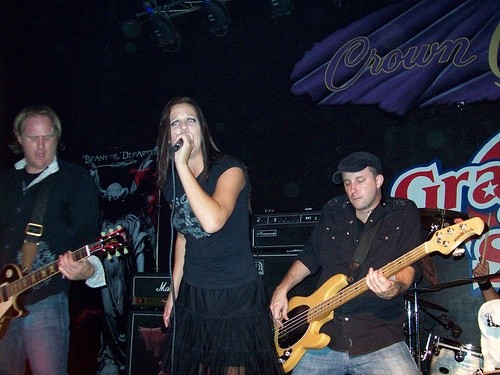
332 152 381 184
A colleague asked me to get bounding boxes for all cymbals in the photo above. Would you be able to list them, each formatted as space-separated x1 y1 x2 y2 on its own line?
404 293 450 314
418 208 489 236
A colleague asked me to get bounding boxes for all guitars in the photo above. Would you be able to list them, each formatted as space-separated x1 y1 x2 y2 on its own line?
1 224 134 340
273 215 485 374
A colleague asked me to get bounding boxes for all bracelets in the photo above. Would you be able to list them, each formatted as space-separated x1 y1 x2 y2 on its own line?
478 280 492 291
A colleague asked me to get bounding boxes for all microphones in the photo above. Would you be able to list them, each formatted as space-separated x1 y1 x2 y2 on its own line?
445 315 462 338
173 139 183 151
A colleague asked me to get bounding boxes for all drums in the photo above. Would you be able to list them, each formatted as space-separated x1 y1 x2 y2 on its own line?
426 334 484 374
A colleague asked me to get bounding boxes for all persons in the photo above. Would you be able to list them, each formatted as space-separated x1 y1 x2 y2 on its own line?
157 96 285 375
270 152 422 375
472 258 500 302
0 104 109 375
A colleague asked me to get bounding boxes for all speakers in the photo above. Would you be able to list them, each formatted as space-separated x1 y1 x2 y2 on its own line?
254 252 322 310
126 311 172 375
